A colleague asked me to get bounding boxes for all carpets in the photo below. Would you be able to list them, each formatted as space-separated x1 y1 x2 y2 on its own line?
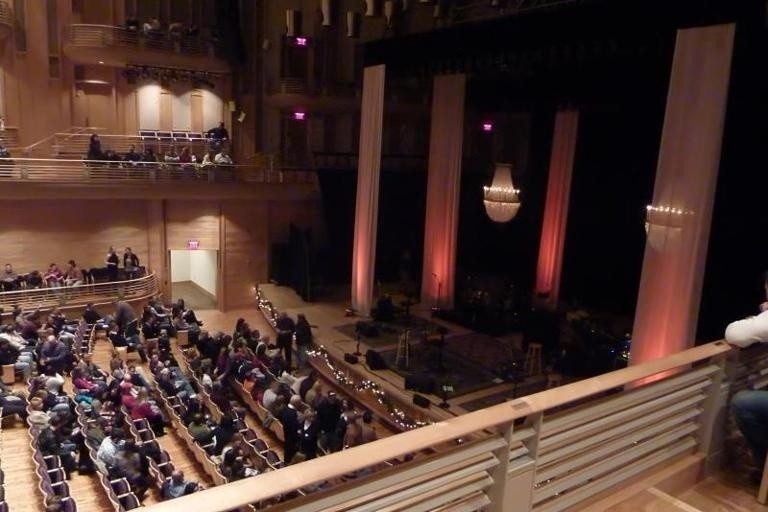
331 313 623 434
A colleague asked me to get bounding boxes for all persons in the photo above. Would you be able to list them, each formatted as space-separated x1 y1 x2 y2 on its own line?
123 246 140 294
44 262 63 298
120 12 229 64
24 270 46 298
61 259 85 298
1 263 20 298
206 119 229 146
725 275 767 484
104 245 121 295
88 132 235 180
1 295 377 512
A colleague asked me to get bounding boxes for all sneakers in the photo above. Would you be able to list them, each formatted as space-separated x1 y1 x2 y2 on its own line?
749 469 762 484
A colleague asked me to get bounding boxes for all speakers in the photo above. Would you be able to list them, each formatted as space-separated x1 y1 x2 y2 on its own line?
413 394 429 408
405 374 434 387
357 320 378 337
344 352 357 365
366 350 386 370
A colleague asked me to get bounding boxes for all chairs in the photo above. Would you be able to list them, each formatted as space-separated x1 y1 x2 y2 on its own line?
137 128 216 143
0 265 149 304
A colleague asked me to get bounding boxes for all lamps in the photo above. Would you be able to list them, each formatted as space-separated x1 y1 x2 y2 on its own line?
643 183 696 260
478 158 524 225
